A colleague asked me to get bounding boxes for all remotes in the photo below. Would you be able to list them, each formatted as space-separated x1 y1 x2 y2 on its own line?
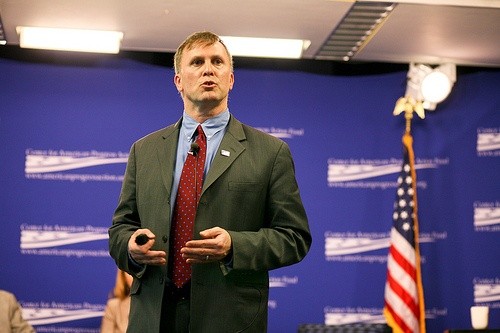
136 233 148 244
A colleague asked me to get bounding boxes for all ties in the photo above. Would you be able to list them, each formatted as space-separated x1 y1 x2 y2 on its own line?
172 125 207 289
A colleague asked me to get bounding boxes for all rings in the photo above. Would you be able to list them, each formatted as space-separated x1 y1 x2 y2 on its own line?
206 256 209 261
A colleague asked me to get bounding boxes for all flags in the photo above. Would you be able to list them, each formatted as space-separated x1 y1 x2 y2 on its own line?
383 144 421 333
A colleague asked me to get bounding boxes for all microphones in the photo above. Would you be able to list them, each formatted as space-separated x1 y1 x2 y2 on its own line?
191 143 199 152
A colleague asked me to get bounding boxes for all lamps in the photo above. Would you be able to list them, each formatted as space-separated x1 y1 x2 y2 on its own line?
218 36 311 60
16 26 124 54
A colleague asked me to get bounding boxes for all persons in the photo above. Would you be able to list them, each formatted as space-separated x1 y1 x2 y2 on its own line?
102 266 133 333
108 31 312 333
0 290 36 333
